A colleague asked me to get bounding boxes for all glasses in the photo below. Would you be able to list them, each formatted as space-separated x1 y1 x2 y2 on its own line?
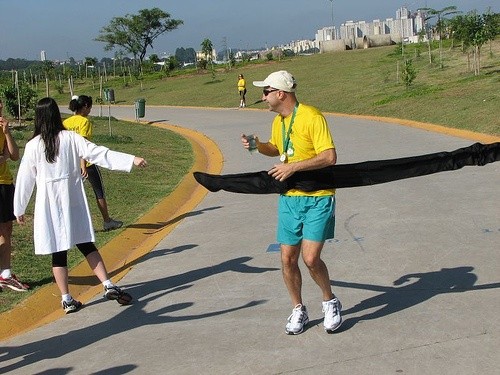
263 89 278 96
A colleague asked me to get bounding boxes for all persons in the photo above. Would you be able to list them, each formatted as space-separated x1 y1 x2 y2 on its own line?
241 71 342 333
238 74 247 107
14 97 147 314
63 95 122 229
0 100 30 292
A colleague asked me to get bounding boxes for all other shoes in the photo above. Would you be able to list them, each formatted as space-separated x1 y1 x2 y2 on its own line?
239 103 246 108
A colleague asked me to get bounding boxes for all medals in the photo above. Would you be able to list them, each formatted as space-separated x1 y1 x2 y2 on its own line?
279 154 288 162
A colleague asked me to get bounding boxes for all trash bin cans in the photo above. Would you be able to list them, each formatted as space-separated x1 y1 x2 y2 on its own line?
108 89 115 101
104 88 110 101
135 98 146 118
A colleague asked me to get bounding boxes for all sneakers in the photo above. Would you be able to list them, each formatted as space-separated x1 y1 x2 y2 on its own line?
322 298 342 332
0 274 29 292
62 296 82 313
105 285 132 304
286 304 308 335
104 218 123 230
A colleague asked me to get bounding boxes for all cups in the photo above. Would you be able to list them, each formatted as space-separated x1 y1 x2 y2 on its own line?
245 133 259 155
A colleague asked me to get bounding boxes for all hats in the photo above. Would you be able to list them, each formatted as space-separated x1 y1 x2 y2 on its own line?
253 70 297 92
